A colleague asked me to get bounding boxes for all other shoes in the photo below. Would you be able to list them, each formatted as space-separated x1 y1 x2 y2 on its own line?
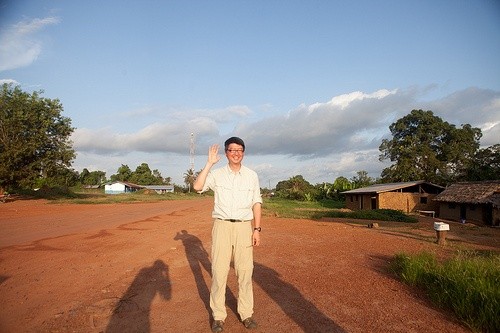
211 320 224 333
243 317 257 329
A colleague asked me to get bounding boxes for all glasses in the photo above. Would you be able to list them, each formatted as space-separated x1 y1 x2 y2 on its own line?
226 148 243 153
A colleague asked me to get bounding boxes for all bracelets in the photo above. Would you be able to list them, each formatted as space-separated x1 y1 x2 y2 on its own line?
254 227 261 232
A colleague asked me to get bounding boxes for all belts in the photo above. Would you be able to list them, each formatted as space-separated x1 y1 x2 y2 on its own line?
217 218 249 222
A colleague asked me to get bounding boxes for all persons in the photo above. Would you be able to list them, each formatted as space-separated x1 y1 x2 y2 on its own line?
193 137 263 333
